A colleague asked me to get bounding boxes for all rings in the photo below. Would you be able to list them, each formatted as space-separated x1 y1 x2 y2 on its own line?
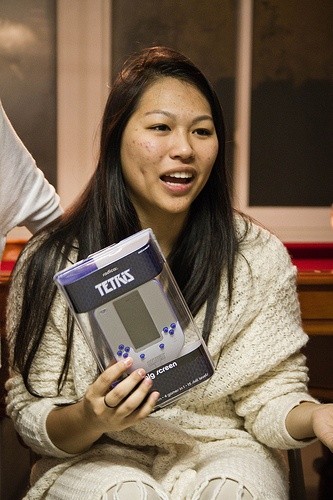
104 395 118 408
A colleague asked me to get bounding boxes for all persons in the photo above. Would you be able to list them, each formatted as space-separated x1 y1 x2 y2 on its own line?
0 99 67 268
5 47 333 500
